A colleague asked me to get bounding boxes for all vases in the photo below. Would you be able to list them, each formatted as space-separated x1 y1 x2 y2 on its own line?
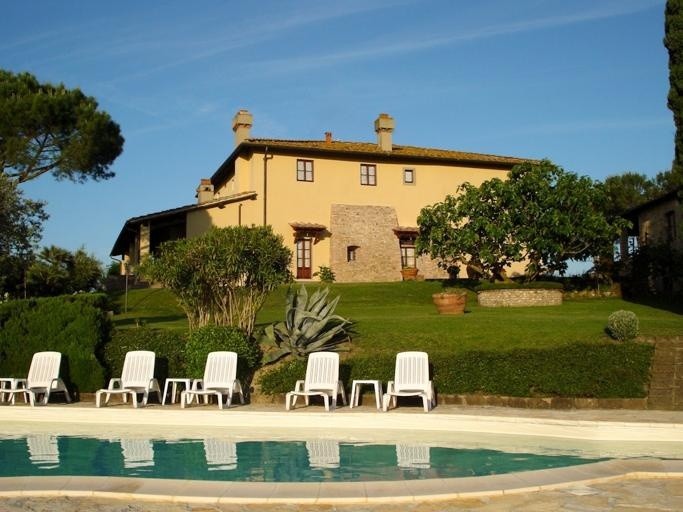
400 267 419 281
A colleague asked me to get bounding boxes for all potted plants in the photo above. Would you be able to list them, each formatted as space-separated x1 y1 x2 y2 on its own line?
432 287 467 314
447 265 460 279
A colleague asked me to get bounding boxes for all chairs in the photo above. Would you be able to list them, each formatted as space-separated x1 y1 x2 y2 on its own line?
120 439 155 468
96 350 245 409
0 351 71 406
306 441 340 470
395 444 431 470
203 439 238 471
27 436 62 470
285 350 436 412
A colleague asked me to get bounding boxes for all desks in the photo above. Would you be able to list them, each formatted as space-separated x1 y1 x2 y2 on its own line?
0 378 28 405
